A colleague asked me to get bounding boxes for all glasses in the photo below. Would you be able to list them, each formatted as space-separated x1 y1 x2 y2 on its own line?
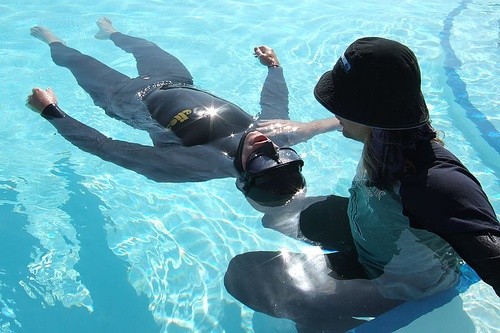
245 147 301 171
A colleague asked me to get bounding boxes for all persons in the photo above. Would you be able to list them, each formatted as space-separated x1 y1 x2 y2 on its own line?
222 38 500 332
27 16 306 212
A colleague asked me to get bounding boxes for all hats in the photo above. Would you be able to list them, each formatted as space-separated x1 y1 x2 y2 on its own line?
235 159 308 208
318 37 430 129
